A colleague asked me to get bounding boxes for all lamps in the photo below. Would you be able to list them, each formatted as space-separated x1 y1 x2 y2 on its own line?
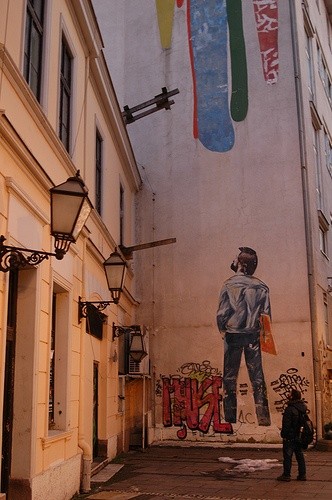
78 247 129 325
112 322 148 365
0 169 95 273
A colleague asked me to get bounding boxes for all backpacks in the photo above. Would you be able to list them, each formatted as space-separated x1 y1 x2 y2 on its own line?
296 413 314 449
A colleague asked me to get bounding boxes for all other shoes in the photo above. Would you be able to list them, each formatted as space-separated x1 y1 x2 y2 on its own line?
297 475 306 481
277 474 290 481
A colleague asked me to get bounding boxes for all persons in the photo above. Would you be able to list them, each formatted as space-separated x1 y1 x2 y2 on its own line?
275 390 308 482
